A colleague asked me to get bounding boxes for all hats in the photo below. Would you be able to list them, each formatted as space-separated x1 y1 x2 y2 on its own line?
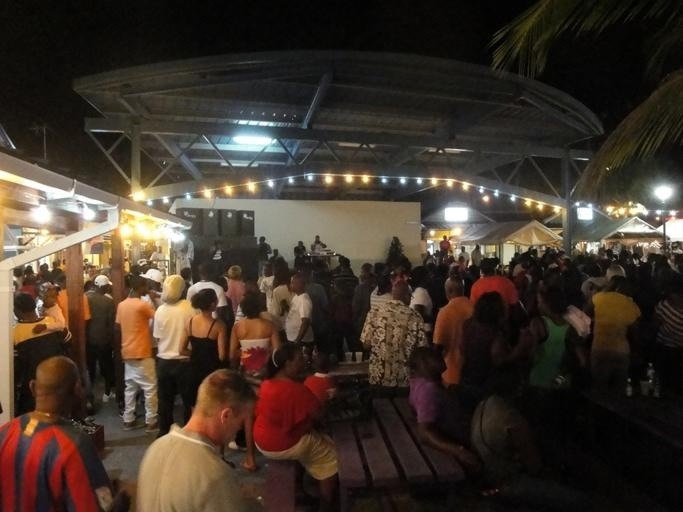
94 274 112 288
39 281 60 300
139 269 162 283
606 265 626 282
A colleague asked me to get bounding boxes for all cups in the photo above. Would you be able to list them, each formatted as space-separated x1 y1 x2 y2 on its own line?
354 351 362 363
345 351 353 363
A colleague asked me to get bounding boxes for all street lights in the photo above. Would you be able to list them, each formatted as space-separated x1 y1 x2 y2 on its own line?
655 185 672 256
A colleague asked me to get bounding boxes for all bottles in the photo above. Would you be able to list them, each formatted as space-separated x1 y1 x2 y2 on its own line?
624 362 660 398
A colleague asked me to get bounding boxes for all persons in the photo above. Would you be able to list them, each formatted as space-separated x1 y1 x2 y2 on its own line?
0 235 682 512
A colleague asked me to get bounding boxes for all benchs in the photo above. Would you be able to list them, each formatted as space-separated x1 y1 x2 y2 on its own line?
258 455 305 510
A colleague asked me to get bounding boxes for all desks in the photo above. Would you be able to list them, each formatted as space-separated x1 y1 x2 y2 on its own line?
324 395 469 509
324 362 368 391
573 369 681 465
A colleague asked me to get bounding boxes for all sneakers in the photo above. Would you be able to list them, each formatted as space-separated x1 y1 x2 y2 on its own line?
123 421 146 431
228 441 247 452
102 392 116 402
145 424 160 433
240 459 257 470
